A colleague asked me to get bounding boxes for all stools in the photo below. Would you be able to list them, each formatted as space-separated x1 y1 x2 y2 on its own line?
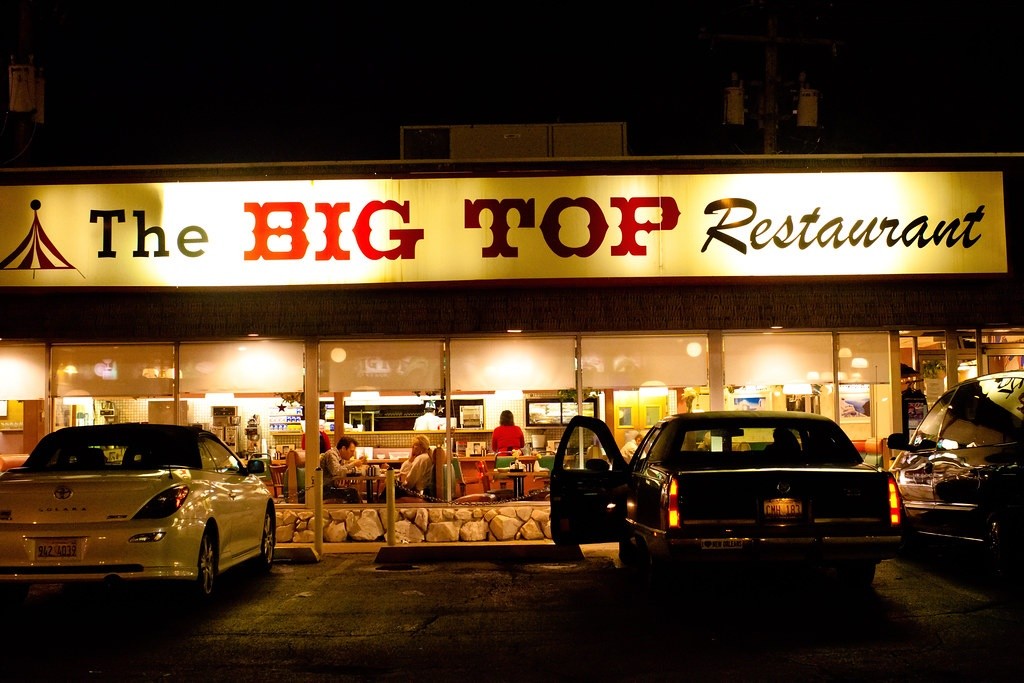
452 453 479 497
496 451 515 488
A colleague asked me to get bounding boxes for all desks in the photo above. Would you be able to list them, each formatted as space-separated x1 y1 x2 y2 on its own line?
339 476 385 504
492 471 549 502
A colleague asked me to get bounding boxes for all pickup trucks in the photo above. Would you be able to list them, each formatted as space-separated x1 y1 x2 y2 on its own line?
550 410 904 607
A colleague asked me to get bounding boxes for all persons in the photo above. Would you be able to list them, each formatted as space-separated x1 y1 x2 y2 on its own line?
413 401 445 430
620 433 644 464
491 410 525 457
377 435 433 503
319 436 367 504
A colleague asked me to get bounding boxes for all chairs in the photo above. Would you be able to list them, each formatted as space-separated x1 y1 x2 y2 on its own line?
76 448 106 469
268 464 288 497
250 453 272 484
476 460 513 498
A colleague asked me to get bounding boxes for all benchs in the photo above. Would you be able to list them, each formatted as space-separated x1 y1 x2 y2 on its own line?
273 452 344 503
395 450 496 502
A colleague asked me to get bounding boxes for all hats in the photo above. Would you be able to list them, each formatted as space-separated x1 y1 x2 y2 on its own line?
425 401 436 410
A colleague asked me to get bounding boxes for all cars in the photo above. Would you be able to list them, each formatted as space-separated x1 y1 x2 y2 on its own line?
0 423 275 605
886 371 1024 595
